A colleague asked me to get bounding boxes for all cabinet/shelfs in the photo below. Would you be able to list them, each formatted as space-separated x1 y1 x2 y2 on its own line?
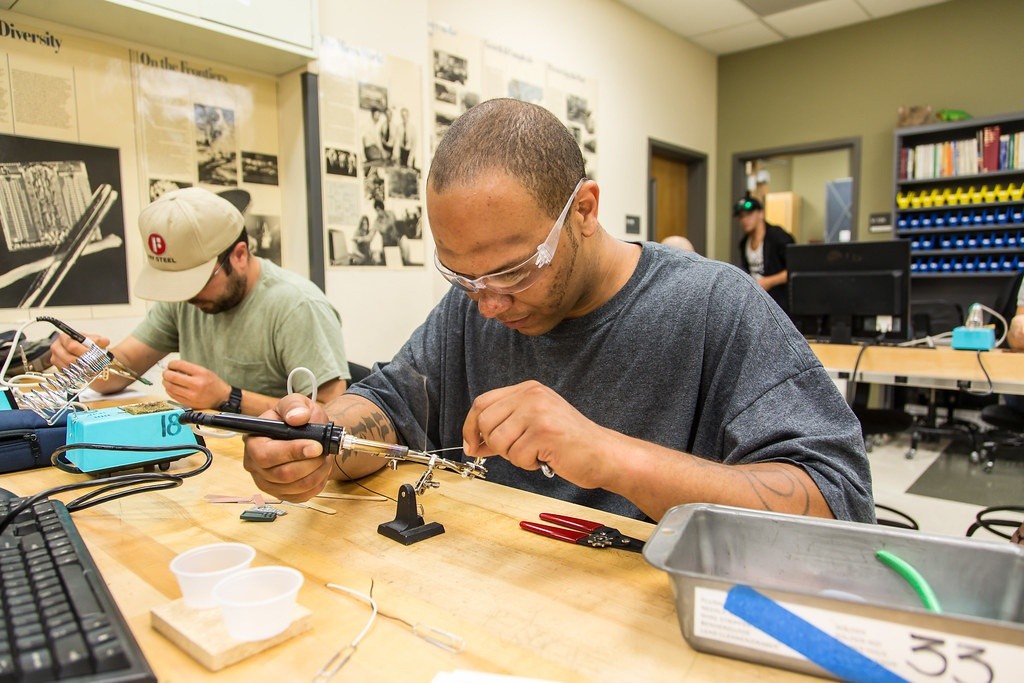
891 112 1024 278
0 0 321 75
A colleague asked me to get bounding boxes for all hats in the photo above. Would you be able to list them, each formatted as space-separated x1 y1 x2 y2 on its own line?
134 187 244 303
732 197 762 216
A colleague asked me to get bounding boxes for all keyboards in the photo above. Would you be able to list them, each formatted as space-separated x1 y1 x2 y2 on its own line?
0 496 159 683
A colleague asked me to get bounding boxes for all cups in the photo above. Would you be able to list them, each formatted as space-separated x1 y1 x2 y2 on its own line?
169 542 256 609
213 565 303 641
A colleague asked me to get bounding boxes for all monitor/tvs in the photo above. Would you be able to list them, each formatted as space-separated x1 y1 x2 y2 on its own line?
786 240 912 347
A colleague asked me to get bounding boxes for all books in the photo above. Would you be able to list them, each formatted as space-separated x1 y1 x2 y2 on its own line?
899 125 1024 181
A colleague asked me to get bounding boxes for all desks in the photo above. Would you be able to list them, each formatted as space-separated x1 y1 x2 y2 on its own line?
801 343 1023 395
0 374 848 683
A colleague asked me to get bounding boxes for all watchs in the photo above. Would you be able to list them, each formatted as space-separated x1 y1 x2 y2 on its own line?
217 383 242 414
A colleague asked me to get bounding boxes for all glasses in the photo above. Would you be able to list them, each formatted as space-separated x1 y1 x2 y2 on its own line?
433 176 584 295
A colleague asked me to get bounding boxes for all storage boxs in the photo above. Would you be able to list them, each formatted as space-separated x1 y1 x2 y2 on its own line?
640 501 1023 683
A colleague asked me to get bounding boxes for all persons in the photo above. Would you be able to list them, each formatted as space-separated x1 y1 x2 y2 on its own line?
49 186 348 418
331 200 401 266
733 196 794 316
1007 273 1024 350
661 235 695 256
241 97 876 525
361 105 415 166
